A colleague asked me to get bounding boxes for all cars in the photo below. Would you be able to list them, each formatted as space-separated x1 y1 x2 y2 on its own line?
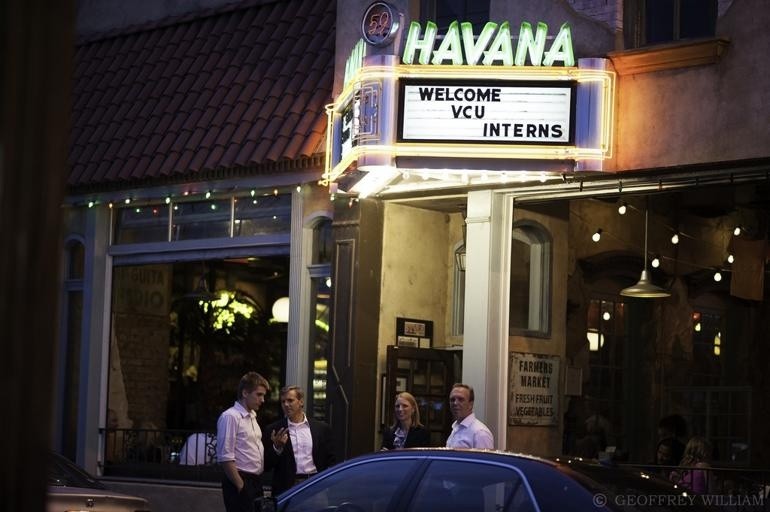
244 445 626 511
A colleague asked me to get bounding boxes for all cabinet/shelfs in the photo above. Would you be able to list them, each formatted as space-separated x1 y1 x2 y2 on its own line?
664 386 753 467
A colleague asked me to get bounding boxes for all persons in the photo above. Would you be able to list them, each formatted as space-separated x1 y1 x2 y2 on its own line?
262 383 347 500
563 386 736 500
440 382 495 450
106 407 120 463
378 392 432 452
178 422 217 465
216 370 271 512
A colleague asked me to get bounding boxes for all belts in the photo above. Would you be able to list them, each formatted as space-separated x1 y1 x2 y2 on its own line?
297 473 318 478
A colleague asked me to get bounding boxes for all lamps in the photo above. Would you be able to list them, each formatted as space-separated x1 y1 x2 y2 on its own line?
455 209 465 272
591 195 741 298
